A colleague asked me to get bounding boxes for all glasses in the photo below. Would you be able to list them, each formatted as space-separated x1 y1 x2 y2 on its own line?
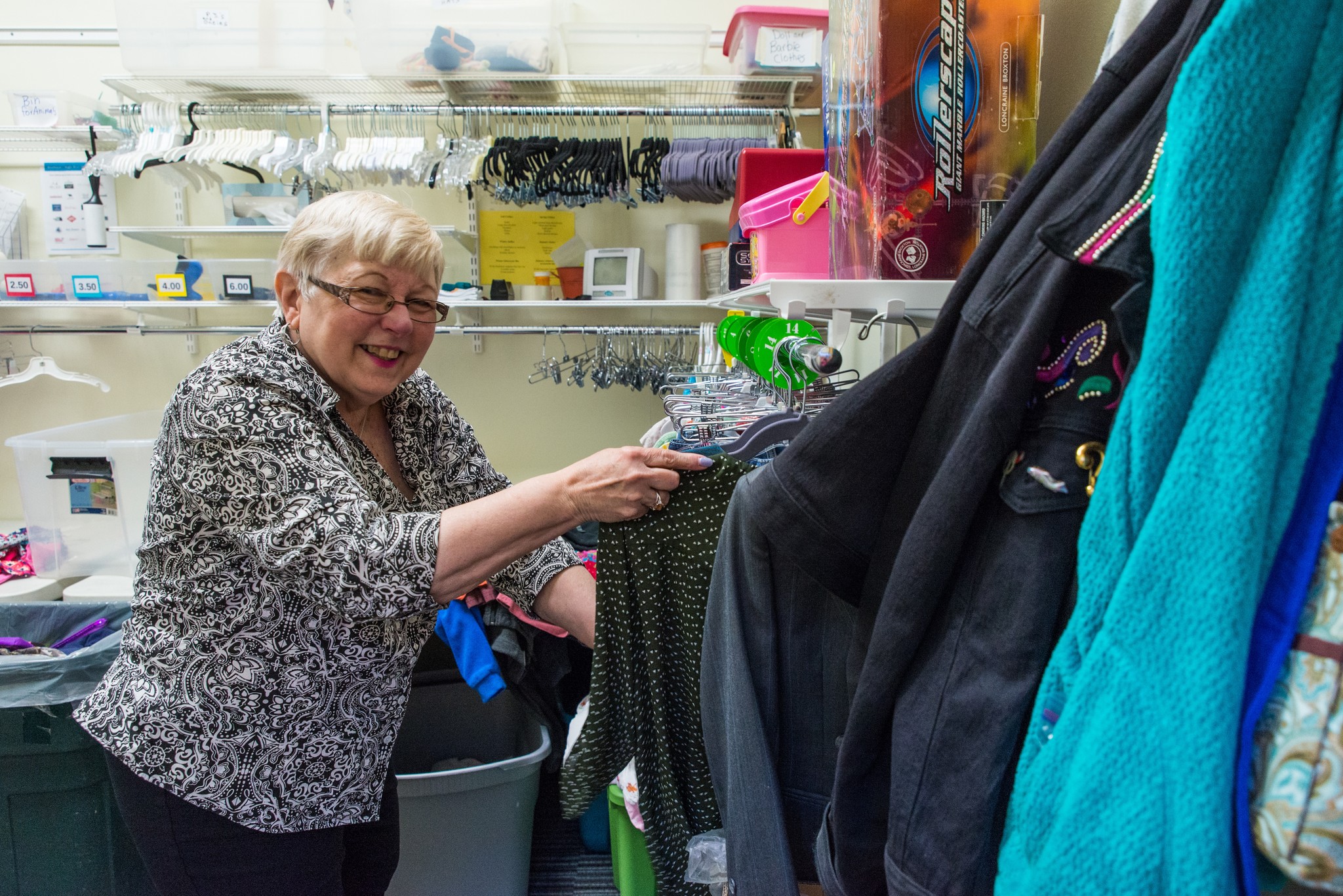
298 270 449 324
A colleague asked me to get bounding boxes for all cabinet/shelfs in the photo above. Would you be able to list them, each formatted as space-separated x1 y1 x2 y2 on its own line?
1 73 811 308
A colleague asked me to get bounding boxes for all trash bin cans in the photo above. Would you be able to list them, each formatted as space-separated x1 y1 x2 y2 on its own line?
388 677 551 896
0 601 154 896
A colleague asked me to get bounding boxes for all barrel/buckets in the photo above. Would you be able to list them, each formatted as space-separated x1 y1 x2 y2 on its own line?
556 266 583 300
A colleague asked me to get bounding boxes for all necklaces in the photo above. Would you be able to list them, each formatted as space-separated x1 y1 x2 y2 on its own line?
360 406 367 440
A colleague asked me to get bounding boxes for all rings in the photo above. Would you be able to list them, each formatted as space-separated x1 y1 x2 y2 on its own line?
649 488 664 510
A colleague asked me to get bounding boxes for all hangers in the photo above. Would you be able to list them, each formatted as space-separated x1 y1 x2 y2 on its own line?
525 313 923 457
83 102 790 205
0 326 113 397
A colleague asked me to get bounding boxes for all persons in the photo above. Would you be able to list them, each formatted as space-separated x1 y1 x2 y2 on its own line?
72 186 718 896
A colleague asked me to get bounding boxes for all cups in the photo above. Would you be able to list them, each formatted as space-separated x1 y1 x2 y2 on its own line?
534 271 549 285
700 241 729 294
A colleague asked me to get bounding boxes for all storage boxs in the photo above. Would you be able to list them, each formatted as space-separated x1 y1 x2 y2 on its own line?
720 243 750 293
824 0 1043 281
722 6 828 98
0 258 279 303
5 410 165 578
739 173 829 284
385 685 554 896
606 784 654 896
